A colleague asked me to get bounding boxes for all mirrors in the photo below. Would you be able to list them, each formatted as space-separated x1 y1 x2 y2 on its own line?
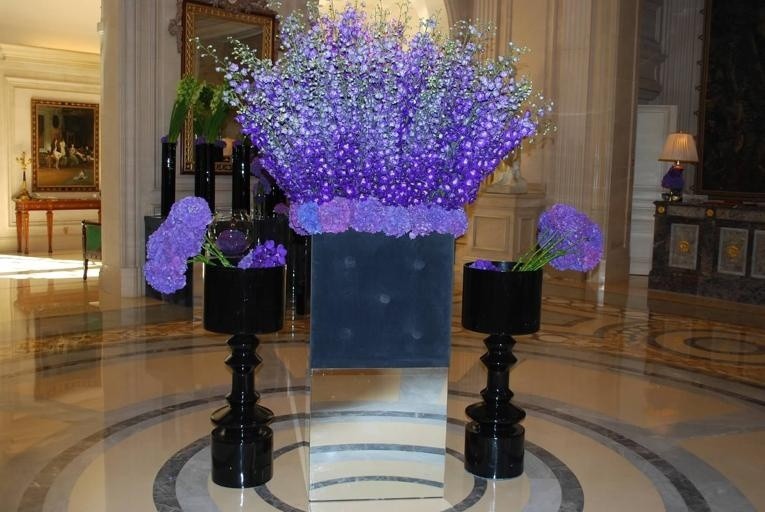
181 3 283 178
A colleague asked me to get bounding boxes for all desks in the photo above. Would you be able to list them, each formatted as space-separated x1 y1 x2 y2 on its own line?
644 192 764 314
10 196 101 254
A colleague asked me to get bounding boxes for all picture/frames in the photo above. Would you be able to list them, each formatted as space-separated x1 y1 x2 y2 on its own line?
29 95 100 202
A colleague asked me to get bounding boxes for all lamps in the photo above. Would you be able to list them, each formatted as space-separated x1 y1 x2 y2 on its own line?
657 129 699 199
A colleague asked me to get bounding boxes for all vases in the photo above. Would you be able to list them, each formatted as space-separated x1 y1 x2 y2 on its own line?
306 233 455 500
204 232 290 489
11 172 32 203
462 231 543 481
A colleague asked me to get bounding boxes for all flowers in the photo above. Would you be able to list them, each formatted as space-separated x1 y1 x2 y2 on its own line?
15 151 34 171
103 0 607 299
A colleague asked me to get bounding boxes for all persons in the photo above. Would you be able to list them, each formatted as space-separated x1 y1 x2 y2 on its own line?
50 137 91 170
